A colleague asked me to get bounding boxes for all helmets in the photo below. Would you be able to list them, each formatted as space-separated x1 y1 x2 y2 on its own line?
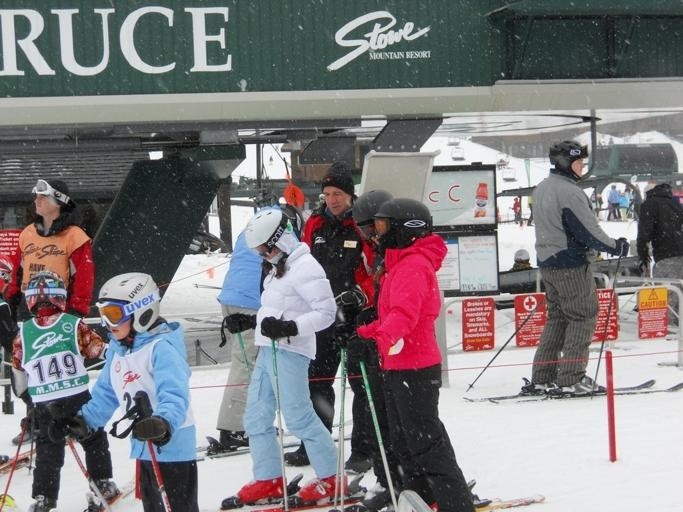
245 209 293 256
96 272 161 334
550 139 589 166
24 271 68 315
352 188 396 227
373 197 434 249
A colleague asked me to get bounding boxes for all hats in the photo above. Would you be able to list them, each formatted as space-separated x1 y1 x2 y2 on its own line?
514 249 530 261
321 161 355 196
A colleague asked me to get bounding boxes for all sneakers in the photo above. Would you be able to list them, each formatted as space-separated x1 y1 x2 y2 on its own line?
219 428 311 468
12 429 40 445
298 473 348 501
28 495 58 512
345 452 373 475
531 373 561 391
561 375 605 395
87 480 119 511
237 475 287 504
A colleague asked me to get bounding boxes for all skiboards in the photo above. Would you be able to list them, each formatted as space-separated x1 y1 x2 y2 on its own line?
202 481 386 512
194 416 352 462
330 479 545 512
462 378 683 405
0 448 36 475
82 476 136 512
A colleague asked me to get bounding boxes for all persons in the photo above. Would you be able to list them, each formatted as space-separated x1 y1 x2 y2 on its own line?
216 161 476 511
513 139 682 395
49 273 198 511
0 179 119 511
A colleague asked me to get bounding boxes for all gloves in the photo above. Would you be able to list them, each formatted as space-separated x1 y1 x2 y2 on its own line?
346 334 376 365
334 285 370 316
260 316 299 341
225 312 257 335
132 416 172 447
607 237 631 257
48 414 88 444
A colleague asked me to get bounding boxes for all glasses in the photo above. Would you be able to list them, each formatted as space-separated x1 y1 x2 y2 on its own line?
32 179 71 205
